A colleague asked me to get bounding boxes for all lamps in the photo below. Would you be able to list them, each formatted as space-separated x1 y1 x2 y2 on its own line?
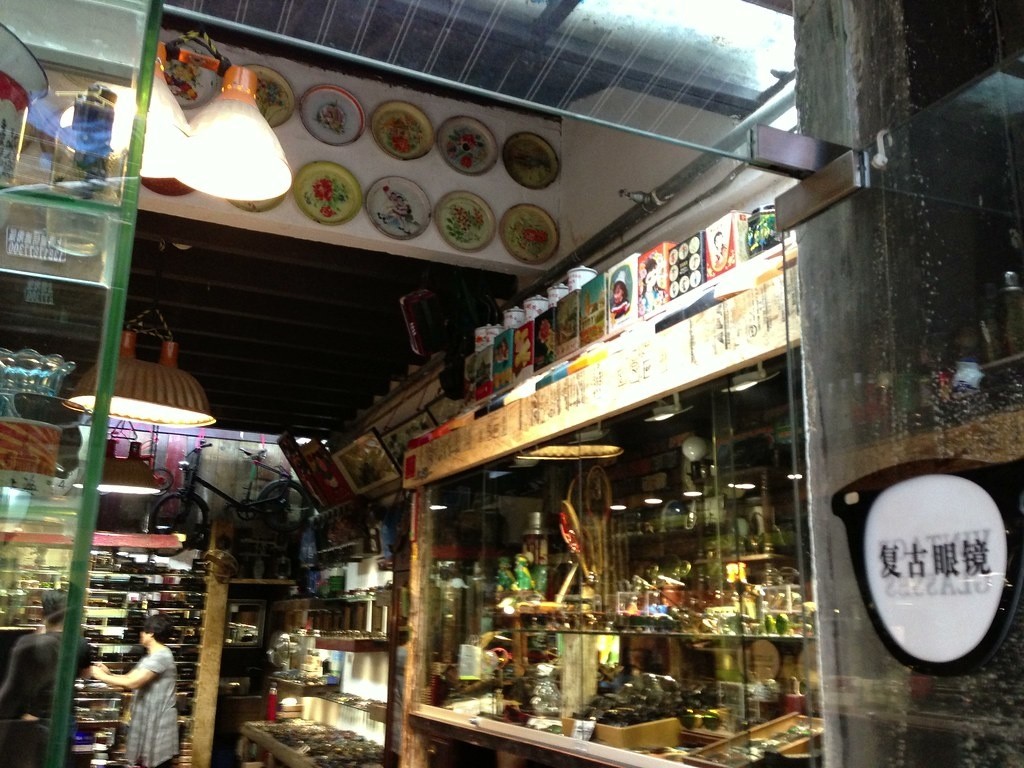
720 361 782 393
138 28 293 201
63 302 216 428
566 422 611 443
643 393 694 422
508 446 539 467
96 418 161 495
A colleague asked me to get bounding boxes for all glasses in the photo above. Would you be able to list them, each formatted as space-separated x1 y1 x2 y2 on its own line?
307 628 385 638
521 610 812 638
480 646 819 768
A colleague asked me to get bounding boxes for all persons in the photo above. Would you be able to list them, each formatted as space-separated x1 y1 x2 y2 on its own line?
80 614 179 768
289 448 341 489
611 270 631 319
0 590 92 768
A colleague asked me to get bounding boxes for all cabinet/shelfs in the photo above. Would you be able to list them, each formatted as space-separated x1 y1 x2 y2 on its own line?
239 632 389 767
1 0 162 767
217 583 271 698
1 530 210 768
399 264 821 767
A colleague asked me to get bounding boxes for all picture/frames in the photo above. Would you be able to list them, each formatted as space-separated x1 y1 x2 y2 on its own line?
327 428 402 497
423 394 462 426
378 410 439 476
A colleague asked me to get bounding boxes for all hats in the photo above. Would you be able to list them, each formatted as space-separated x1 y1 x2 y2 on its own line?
42 589 67 616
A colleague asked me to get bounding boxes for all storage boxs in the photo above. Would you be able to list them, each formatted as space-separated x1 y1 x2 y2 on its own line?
512 320 534 383
556 288 580 360
704 210 750 283
494 329 514 393
534 307 555 371
580 270 608 347
667 228 706 302
608 251 640 333
637 242 678 318
463 345 493 404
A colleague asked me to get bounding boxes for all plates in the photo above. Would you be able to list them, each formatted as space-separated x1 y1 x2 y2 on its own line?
366 176 432 240
372 100 434 161
227 194 286 212
141 177 196 196
165 44 223 108
499 203 559 264
502 132 559 189
298 85 365 147
242 63 294 128
434 190 497 253
291 160 361 225
437 115 499 176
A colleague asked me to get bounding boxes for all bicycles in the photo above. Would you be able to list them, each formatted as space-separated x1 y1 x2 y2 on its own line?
147 441 317 559
